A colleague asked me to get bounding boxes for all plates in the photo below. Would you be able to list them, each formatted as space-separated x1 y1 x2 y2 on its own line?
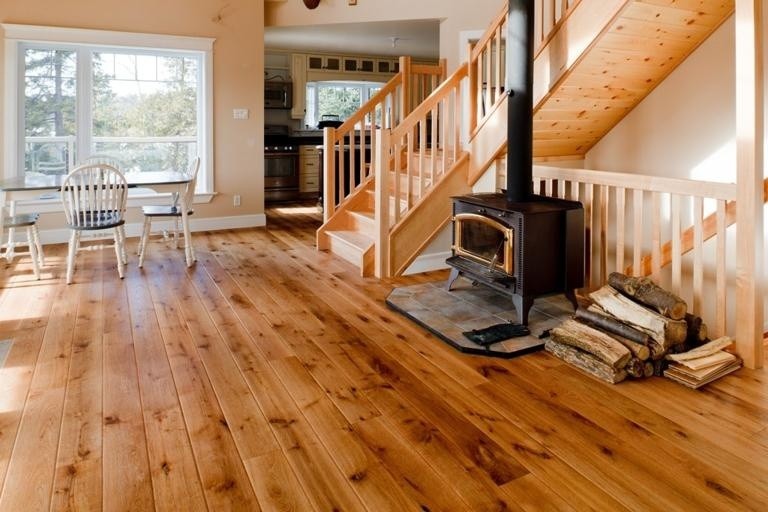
291 52 307 121
390 58 439 123
343 55 375 74
374 58 403 75
307 53 343 73
298 144 321 194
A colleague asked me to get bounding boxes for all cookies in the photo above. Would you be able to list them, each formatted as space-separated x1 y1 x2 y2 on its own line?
264 80 292 109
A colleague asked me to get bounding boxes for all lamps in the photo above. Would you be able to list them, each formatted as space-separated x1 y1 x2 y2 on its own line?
136 155 201 270
67 154 128 242
57 161 132 286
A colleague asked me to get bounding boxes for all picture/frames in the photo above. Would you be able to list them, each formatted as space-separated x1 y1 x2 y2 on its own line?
0 169 195 270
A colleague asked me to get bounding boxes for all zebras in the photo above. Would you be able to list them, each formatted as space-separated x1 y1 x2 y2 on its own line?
263 152 301 202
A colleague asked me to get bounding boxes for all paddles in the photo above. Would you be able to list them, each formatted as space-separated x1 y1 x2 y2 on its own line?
384 275 582 360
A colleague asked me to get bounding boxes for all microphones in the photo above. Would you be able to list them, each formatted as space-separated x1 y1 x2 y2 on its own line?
0 211 45 282
